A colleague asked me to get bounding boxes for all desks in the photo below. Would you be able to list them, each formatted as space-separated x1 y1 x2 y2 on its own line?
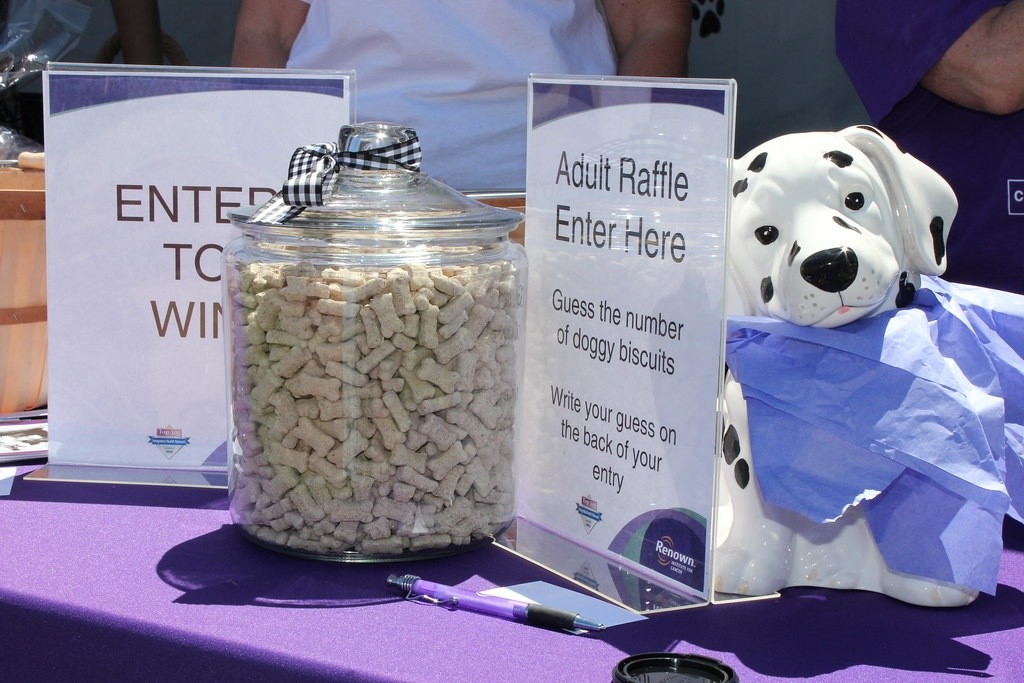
0 460 1024 683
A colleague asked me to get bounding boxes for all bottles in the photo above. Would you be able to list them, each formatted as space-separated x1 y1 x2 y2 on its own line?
223 121 527 562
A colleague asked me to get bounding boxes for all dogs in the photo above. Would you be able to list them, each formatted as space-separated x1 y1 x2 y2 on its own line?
713 122 997 607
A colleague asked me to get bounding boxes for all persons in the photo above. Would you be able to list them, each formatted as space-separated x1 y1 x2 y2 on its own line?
231 0 690 192
0 0 161 145
834 0 1024 296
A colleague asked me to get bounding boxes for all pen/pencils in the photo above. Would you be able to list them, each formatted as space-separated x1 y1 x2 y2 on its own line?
386 574 606 632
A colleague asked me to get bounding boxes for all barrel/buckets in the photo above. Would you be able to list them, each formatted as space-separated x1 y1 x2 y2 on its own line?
0 153 47 412
